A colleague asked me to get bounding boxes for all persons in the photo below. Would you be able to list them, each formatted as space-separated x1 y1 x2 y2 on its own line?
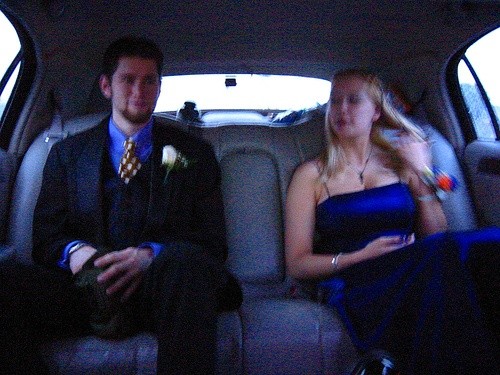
0 36 227 375
284 68 500 375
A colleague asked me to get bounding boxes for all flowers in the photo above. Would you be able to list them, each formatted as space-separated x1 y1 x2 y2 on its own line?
161 145 178 180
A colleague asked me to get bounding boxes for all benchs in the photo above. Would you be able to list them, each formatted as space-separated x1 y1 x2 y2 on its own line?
10 113 483 375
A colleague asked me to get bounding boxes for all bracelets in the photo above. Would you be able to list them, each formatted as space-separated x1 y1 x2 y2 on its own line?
412 193 436 201
332 252 341 272
69 242 88 253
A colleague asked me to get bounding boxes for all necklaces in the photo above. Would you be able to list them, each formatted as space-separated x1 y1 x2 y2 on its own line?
347 144 373 185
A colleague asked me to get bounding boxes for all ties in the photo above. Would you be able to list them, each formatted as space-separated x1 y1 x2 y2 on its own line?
116 138 142 185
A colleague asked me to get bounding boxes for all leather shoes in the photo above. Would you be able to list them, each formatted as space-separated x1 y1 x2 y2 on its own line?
349 358 393 375
